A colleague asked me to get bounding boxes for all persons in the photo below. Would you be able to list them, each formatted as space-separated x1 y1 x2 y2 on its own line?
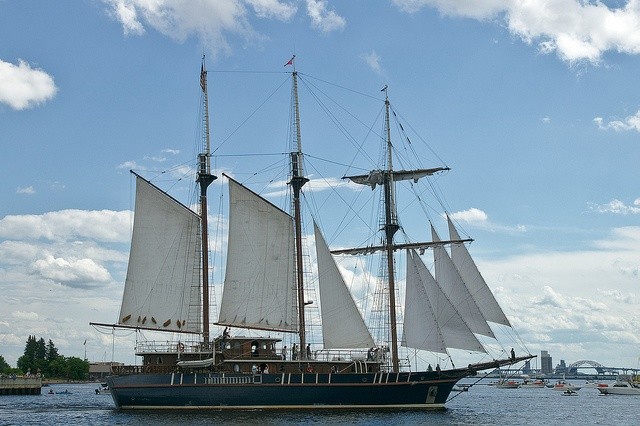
307 343 312 359
282 346 288 360
374 347 380 362
366 347 374 362
146 364 150 373
266 364 269 372
264 367 269 374
380 346 385 362
280 364 284 374
428 363 432 372
296 345 300 360
436 364 440 371
331 365 336 373
510 348 515 359
307 366 312 373
223 327 230 337
252 366 257 374
292 343 296 360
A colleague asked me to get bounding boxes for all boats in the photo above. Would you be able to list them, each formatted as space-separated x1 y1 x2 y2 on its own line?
596 380 640 395
552 381 582 392
496 380 520 388
545 380 554 389
95 384 111 395
561 391 579 396
597 392 614 396
520 379 547 389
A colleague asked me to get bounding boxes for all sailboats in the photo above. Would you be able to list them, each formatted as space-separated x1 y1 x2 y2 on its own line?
88 52 538 410
